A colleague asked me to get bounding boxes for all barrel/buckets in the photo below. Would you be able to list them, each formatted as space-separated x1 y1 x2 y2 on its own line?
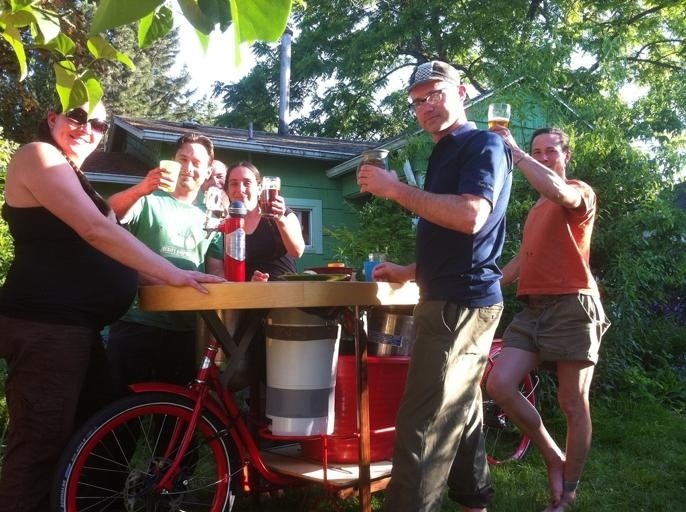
302 357 408 463
261 310 341 435
365 310 413 356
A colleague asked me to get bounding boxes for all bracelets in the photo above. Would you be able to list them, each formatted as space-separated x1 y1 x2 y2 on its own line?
514 152 530 166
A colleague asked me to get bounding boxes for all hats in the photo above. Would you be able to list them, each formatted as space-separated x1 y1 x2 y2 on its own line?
406 60 461 98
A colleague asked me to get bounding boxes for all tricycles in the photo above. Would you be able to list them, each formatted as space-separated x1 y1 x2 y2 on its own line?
47 278 542 511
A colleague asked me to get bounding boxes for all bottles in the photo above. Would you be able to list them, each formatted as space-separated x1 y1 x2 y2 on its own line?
357 149 391 192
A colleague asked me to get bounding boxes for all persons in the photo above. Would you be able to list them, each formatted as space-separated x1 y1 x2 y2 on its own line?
203 160 304 439
0 80 227 511
195 157 233 223
103 133 220 511
352 60 514 512
482 117 612 512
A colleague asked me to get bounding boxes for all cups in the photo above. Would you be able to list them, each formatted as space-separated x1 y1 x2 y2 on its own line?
363 252 388 282
259 175 281 220
157 160 182 194
486 101 511 133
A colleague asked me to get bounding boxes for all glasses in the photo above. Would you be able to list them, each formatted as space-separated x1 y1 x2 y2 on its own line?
407 86 449 114
65 107 109 134
